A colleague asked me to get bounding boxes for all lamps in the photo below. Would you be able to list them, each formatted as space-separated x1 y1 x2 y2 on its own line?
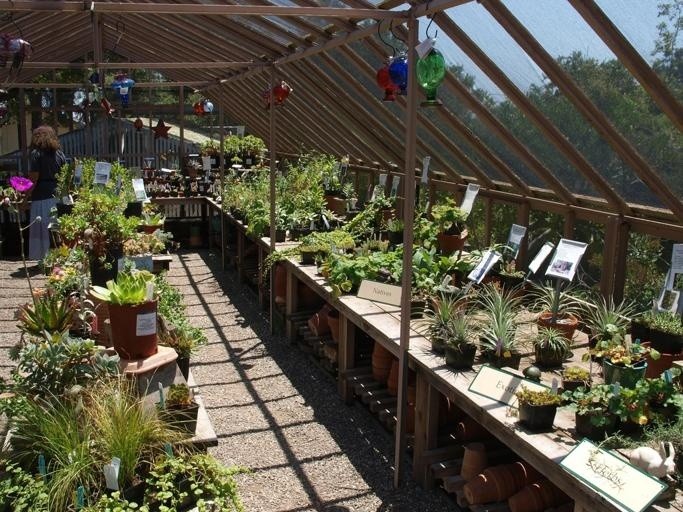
374 3 445 109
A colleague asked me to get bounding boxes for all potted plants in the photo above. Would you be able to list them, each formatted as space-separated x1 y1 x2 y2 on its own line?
51 157 159 360
153 383 200 438
199 132 404 298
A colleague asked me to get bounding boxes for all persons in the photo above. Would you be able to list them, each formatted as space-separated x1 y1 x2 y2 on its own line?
19 125 65 272
